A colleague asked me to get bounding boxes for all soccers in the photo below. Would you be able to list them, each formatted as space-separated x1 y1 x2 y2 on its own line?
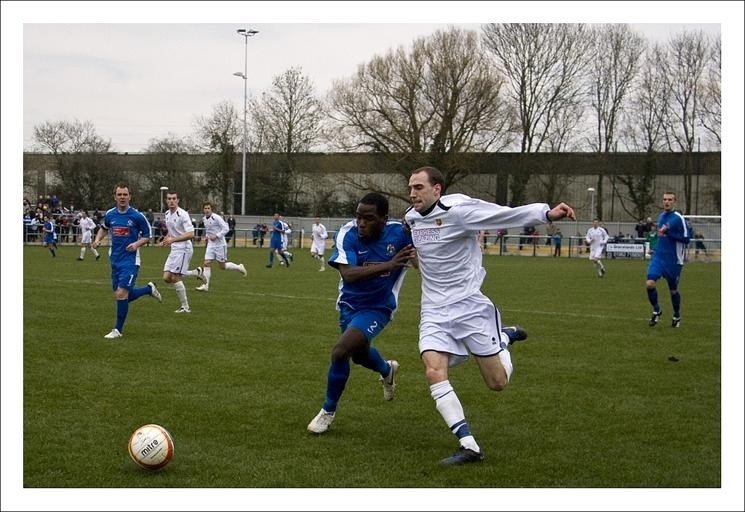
127 422 175 470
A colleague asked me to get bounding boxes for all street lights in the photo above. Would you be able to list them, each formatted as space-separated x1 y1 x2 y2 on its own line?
233 28 260 215
160 186 168 212
587 187 595 227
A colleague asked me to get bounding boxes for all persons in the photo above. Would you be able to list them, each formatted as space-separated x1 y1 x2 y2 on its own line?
608 210 707 262
311 216 327 272
307 192 420 436
23 203 100 261
196 202 248 291
645 190 689 329
578 219 609 278
92 181 161 338
250 213 294 268
144 208 236 243
158 189 208 313
404 165 578 468
482 225 561 256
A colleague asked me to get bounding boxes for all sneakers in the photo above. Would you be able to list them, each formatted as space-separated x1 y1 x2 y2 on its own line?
439 446 485 466
306 407 336 434
672 316 680 328
378 360 399 401
147 281 162 303
173 306 191 313
104 328 122 339
195 263 248 292
501 325 527 345
266 253 294 268
318 267 325 272
649 310 662 326
